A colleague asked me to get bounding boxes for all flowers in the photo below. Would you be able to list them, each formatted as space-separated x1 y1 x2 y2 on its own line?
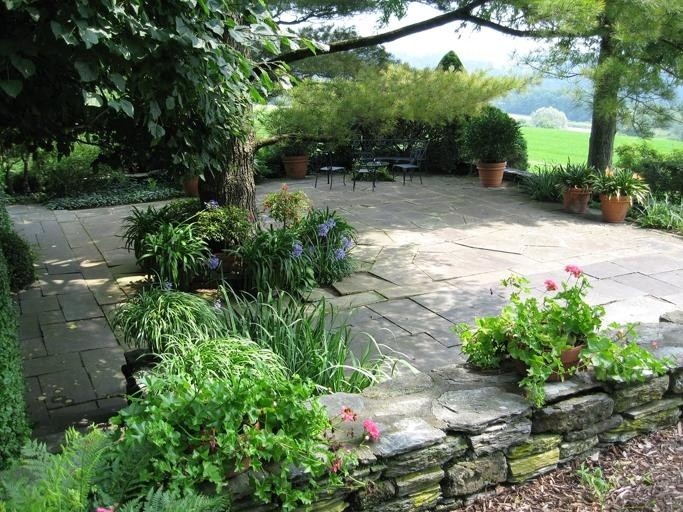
449 267 662 379
109 373 381 512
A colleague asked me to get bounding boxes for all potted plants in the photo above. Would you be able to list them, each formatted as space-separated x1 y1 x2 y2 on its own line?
107 287 168 405
279 127 309 179
462 105 650 223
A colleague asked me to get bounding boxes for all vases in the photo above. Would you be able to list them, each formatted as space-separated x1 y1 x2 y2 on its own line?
188 421 259 479
514 339 588 383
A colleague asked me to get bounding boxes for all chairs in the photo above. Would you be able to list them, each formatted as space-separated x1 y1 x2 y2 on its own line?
314 137 430 192
